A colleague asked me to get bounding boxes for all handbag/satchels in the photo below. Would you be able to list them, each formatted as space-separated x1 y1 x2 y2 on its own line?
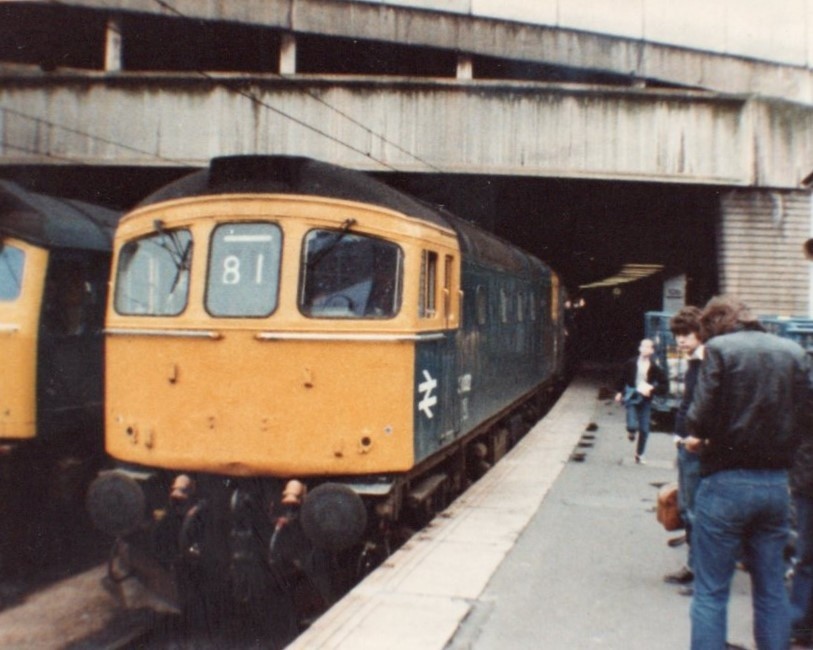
659 486 684 530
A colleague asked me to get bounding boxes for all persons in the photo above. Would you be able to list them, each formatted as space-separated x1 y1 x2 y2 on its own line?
615 338 668 459
662 296 813 650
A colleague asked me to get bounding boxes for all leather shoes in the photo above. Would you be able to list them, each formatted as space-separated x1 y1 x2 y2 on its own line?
664 563 693 584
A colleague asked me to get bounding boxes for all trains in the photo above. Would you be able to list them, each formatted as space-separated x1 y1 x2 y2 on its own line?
86 154 591 629
0 173 125 595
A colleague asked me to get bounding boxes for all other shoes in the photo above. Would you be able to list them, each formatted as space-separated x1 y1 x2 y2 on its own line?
628 431 637 442
634 454 649 466
